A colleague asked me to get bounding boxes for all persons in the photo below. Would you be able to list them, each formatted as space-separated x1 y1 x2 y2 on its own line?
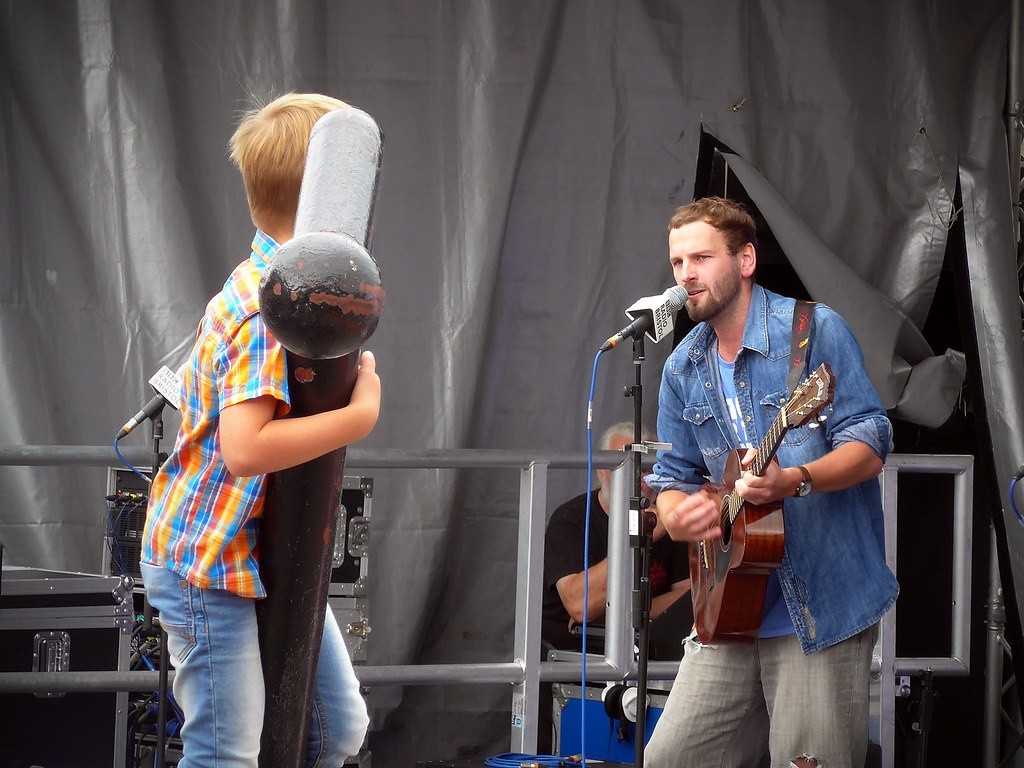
535 419 695 766
136 91 384 763
639 196 908 766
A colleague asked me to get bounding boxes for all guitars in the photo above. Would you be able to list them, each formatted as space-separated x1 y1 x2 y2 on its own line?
686 361 837 645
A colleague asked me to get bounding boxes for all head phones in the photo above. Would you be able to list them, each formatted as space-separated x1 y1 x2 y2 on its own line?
602 645 651 722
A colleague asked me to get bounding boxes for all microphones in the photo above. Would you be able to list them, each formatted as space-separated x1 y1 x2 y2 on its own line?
115 364 186 439
599 285 689 353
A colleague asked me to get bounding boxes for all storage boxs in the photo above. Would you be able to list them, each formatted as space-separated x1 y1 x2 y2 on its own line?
0 566 135 768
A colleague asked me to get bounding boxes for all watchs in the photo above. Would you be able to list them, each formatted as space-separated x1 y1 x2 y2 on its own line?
792 466 815 498
